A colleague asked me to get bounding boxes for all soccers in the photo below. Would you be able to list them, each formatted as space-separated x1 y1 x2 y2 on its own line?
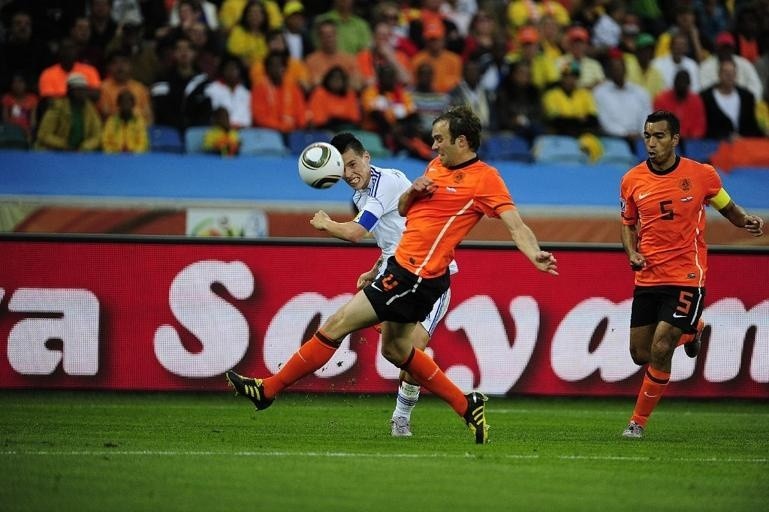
298 141 344 189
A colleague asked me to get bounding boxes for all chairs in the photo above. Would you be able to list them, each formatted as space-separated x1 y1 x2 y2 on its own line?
2 119 768 172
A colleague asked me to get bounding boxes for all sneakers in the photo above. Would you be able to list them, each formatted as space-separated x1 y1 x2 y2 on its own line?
390 416 411 437
623 421 646 439
683 318 705 358
460 390 491 445
225 370 277 410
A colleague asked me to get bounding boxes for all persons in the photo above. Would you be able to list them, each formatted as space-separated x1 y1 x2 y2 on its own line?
225 108 559 444
621 110 765 438
310 134 458 438
2 1 768 157
